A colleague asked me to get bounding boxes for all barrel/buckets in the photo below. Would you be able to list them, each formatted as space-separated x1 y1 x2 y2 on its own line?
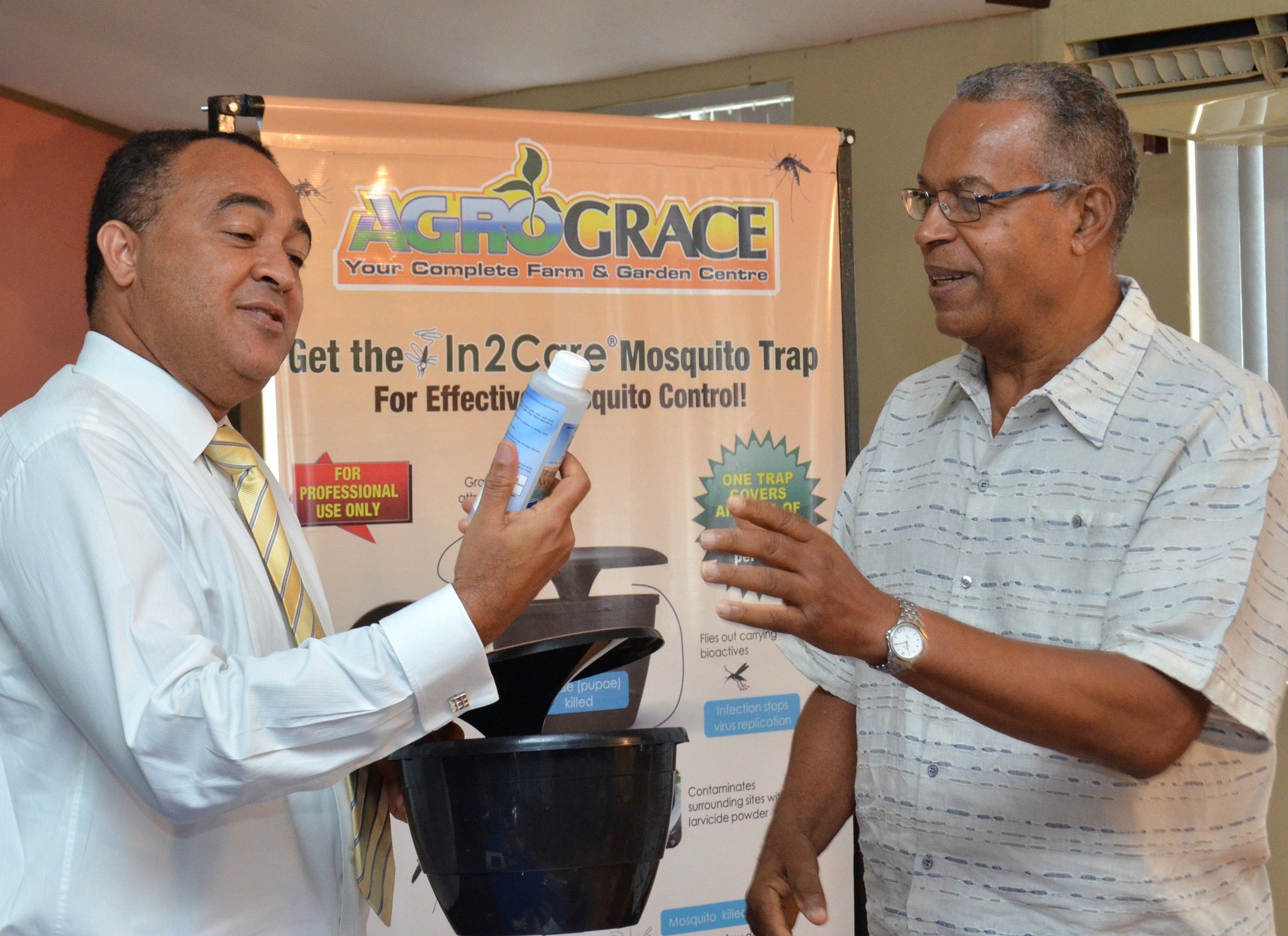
385 726 692 936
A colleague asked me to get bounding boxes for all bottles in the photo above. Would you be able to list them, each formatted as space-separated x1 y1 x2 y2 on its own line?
469 349 592 524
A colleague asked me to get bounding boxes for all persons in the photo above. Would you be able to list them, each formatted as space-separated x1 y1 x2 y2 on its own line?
699 59 1287 936
0 127 592 936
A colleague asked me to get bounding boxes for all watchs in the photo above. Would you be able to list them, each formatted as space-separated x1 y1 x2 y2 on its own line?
865 596 927 674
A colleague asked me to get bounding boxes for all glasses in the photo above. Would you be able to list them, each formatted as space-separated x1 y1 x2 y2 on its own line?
901 177 1083 233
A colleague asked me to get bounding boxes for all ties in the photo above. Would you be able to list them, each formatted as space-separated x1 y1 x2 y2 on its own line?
207 420 400 935
